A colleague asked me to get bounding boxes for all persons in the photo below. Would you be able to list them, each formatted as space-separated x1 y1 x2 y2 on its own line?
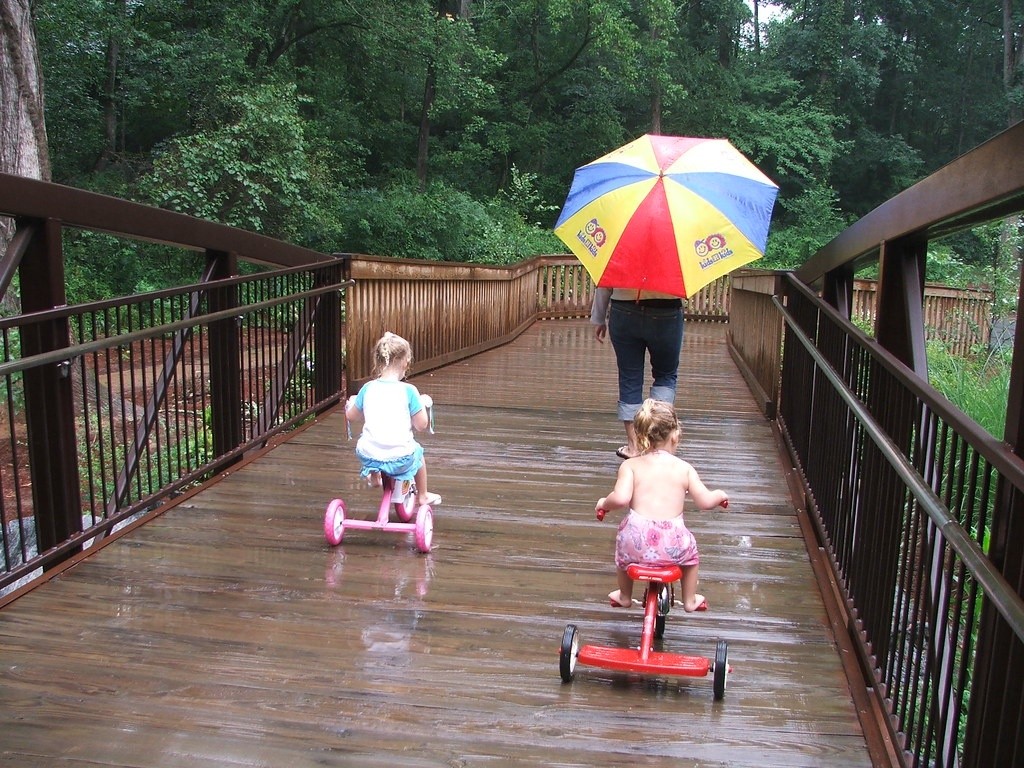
595 398 727 612
345 330 441 507
589 284 683 459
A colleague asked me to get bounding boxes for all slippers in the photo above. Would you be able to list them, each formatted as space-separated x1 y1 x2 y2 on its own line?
616 445 641 460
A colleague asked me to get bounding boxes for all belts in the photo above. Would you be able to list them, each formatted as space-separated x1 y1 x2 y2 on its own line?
611 298 681 305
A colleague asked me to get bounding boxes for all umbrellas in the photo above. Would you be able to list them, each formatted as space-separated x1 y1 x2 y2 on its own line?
552 134 780 301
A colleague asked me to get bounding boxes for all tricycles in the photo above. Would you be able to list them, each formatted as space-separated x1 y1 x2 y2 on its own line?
324 400 433 552
560 501 732 701
